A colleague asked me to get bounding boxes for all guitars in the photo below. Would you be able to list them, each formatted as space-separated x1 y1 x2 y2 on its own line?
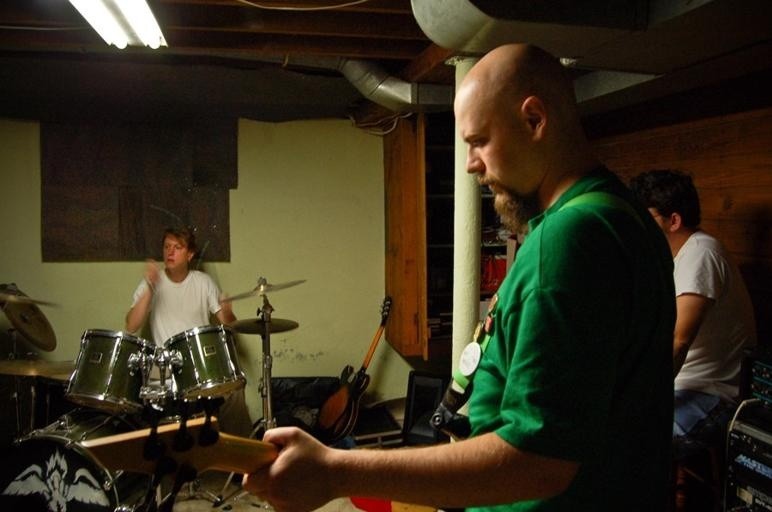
319 296 392 440
82 395 278 511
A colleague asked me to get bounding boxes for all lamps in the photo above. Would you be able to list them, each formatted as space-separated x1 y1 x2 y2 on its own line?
65 0 169 50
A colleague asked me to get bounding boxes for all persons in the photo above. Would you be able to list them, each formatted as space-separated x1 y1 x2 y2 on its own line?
240 40 678 510
125 223 238 349
628 166 759 469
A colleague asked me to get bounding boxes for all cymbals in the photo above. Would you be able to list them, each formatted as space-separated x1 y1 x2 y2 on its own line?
0 284 60 352
221 280 307 303
1 357 73 379
229 319 299 333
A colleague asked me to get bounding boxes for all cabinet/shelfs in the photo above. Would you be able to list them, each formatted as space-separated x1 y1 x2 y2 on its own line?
383 110 508 361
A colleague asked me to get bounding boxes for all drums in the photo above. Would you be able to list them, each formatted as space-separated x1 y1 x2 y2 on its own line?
164 324 247 403
0 407 162 512
65 330 156 413
